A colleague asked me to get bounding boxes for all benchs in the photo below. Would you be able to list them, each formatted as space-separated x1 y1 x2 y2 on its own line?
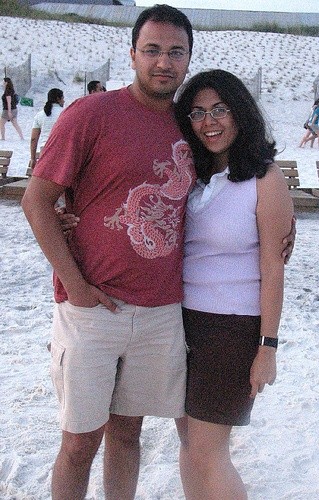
1 150 319 213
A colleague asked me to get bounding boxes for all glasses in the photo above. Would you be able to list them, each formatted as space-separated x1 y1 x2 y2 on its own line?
134 46 190 60
188 107 230 123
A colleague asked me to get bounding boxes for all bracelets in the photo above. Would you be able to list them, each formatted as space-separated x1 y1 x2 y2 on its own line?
258 335 279 349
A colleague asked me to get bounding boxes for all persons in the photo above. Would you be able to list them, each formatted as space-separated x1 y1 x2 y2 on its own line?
296 99 319 149
19 4 296 500
87 81 104 95
50 68 294 500
1 77 26 140
29 88 66 208
